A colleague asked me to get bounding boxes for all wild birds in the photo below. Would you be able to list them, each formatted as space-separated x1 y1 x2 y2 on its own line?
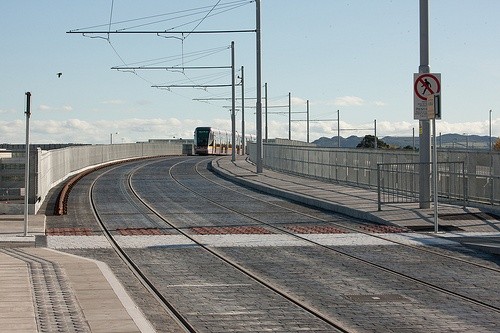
57 73 62 77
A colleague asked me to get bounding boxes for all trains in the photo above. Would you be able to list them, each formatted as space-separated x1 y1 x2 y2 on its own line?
193 127 257 156
